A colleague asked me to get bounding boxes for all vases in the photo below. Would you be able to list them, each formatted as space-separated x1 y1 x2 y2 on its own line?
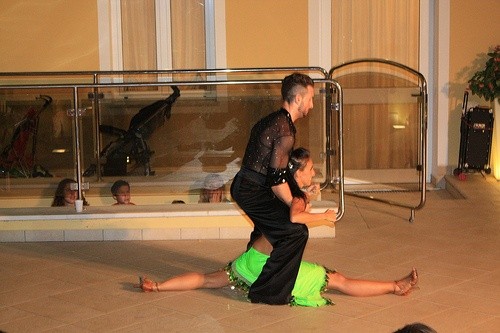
489 98 500 180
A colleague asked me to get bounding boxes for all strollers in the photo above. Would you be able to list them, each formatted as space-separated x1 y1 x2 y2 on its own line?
0 94 57 179
82 84 181 179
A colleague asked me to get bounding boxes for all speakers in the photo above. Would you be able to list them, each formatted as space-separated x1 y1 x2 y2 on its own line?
462 117 493 170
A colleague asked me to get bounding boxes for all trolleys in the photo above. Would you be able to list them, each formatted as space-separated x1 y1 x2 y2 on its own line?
453 91 493 177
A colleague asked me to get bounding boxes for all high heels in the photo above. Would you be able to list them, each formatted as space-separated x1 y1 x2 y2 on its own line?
134 276 159 293
393 265 420 295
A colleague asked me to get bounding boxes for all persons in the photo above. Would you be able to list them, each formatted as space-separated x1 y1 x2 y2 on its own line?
229 72 314 306
392 324 439 333
111 180 136 206
51 178 90 207
197 174 230 203
8 96 71 169
139 147 417 295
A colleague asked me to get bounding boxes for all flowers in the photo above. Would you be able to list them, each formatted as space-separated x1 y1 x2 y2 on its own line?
463 45 500 105
